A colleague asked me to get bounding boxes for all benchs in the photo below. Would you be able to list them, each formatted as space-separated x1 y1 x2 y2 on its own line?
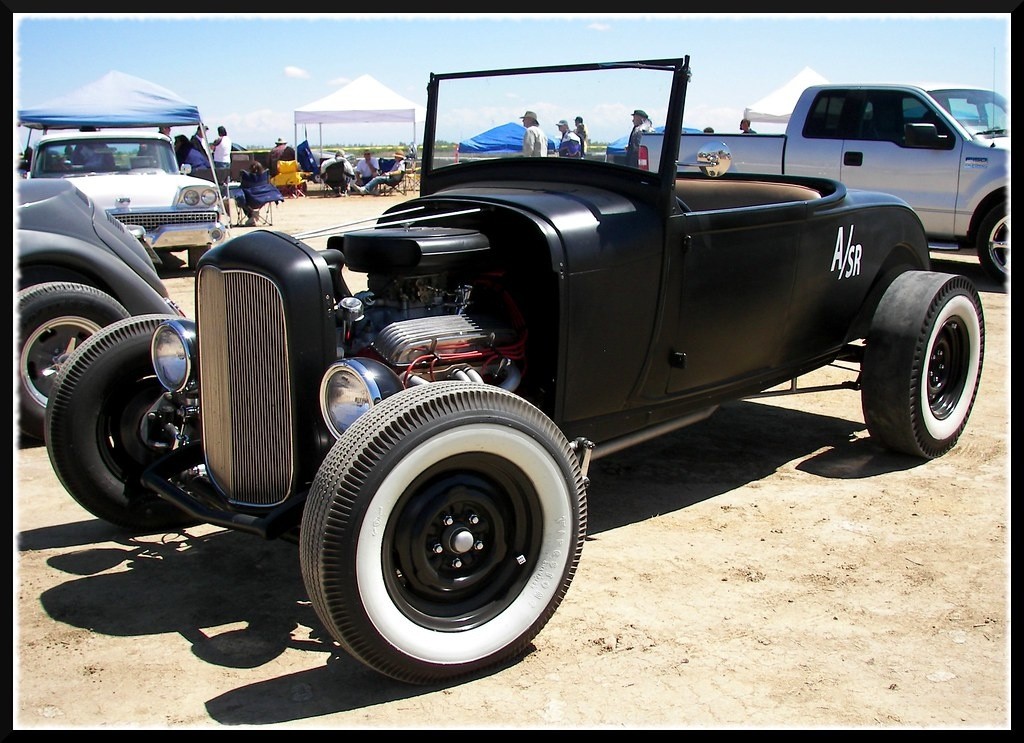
674 176 822 214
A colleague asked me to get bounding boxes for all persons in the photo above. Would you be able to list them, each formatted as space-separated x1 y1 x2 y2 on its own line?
355 150 380 193
556 116 590 158
136 144 148 156
208 143 214 160
519 111 548 157
270 137 304 197
740 119 757 134
245 161 263 226
704 127 714 133
190 125 210 161
355 151 405 195
158 125 173 153
71 126 115 171
321 149 355 196
624 109 655 167
213 125 231 184
174 135 211 176
19 148 34 178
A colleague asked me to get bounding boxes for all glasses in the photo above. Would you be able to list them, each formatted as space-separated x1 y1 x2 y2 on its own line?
365 153 370 155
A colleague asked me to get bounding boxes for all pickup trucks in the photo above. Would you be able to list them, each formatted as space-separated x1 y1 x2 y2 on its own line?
639 83 1008 283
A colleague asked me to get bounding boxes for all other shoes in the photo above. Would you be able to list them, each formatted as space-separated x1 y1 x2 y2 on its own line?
353 184 365 195
246 221 255 227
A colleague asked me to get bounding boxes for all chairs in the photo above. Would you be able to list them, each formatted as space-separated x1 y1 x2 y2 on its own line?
377 158 411 196
320 161 355 199
269 159 313 198
233 169 284 226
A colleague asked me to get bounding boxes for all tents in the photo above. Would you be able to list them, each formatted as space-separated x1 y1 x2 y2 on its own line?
743 66 831 122
17 71 232 238
459 122 563 154
294 75 428 168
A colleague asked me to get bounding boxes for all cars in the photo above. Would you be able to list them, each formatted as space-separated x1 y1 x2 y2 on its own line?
297 149 355 184
19 177 187 437
208 142 247 151
44 56 983 687
29 130 226 269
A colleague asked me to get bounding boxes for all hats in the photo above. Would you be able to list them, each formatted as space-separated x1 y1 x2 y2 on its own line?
520 111 539 126
555 120 568 126
631 110 648 119
198 125 208 131
393 150 406 158
275 137 288 144
